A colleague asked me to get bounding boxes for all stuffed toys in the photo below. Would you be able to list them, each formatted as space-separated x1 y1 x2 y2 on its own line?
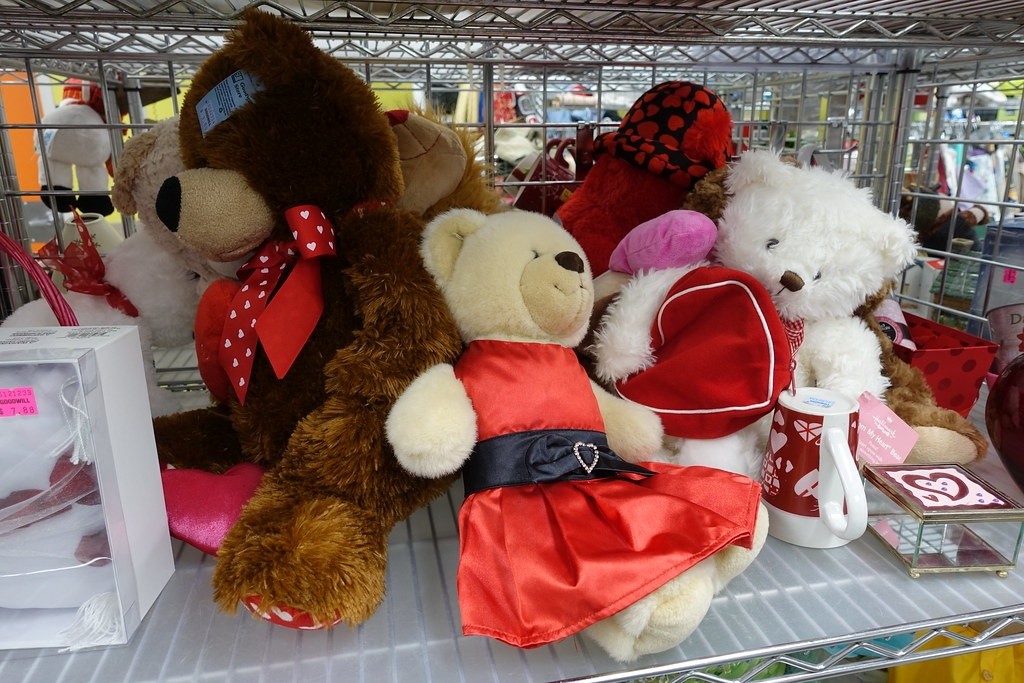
149 10 477 632
2 94 984 467
1 366 113 610
36 74 126 220
383 207 771 664
592 144 918 488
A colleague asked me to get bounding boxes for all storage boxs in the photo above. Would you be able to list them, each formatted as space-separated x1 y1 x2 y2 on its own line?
892 257 942 320
965 219 1024 341
0 323 172 649
919 260 945 319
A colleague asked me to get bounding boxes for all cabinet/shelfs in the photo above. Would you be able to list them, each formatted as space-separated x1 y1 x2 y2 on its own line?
0 0 1024 683
